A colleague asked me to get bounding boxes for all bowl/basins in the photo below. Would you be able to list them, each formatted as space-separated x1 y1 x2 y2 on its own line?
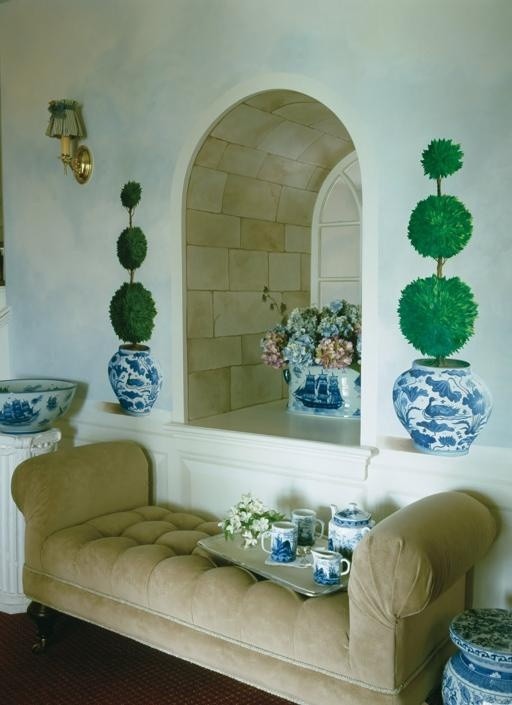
0 377 78 436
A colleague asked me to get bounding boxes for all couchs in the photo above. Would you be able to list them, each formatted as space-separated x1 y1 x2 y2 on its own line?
11 439 499 705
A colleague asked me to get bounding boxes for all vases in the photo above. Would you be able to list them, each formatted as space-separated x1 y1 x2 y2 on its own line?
285 366 360 420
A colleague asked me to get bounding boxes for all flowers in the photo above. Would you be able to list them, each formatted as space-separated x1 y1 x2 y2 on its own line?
259 284 362 370
218 494 283 553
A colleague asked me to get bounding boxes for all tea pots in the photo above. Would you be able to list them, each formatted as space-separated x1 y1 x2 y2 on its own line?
329 503 375 558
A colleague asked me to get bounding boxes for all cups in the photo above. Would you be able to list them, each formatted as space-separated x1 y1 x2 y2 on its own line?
262 522 299 562
291 508 324 546
310 548 351 586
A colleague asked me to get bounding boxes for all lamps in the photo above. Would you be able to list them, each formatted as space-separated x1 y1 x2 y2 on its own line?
44 99 95 187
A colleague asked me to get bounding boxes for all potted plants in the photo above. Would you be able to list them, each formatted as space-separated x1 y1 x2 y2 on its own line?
393 137 494 457
107 180 164 416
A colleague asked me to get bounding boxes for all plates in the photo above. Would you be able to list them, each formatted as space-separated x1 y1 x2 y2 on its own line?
197 529 349 597
